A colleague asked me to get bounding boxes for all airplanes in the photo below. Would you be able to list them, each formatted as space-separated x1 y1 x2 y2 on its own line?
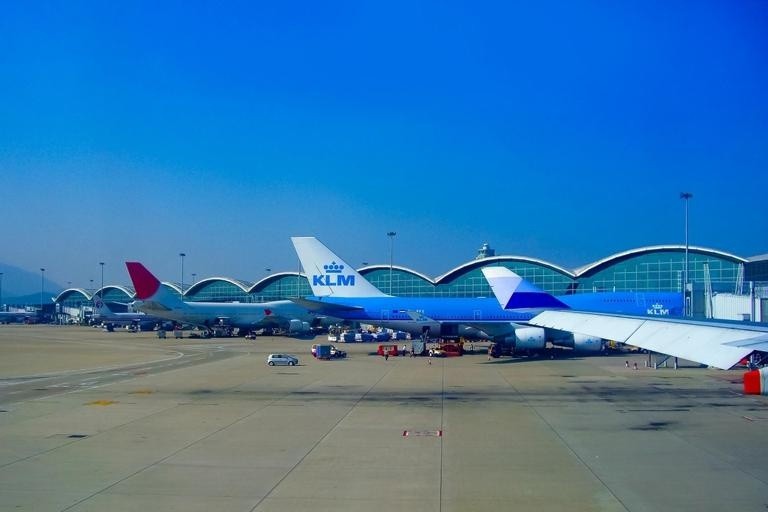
291 236 685 358
125 261 353 336
0 312 53 324
86 294 164 325
480 267 768 394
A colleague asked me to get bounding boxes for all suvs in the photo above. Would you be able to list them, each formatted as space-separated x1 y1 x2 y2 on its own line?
311 343 337 357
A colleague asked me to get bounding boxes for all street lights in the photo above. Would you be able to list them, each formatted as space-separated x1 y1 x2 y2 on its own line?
179 253 186 302
40 262 104 307
680 192 693 282
387 231 396 296
192 272 197 285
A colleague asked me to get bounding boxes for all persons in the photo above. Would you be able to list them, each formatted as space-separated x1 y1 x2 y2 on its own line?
469 343 473 351
409 346 416 358
383 348 388 361
401 345 406 355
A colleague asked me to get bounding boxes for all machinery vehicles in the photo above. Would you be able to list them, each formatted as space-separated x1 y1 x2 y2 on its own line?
487 343 497 355
329 349 346 358
628 345 647 354
429 347 446 357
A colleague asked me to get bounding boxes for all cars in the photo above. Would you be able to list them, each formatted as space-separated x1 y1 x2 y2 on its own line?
244 334 256 340
267 353 298 366
